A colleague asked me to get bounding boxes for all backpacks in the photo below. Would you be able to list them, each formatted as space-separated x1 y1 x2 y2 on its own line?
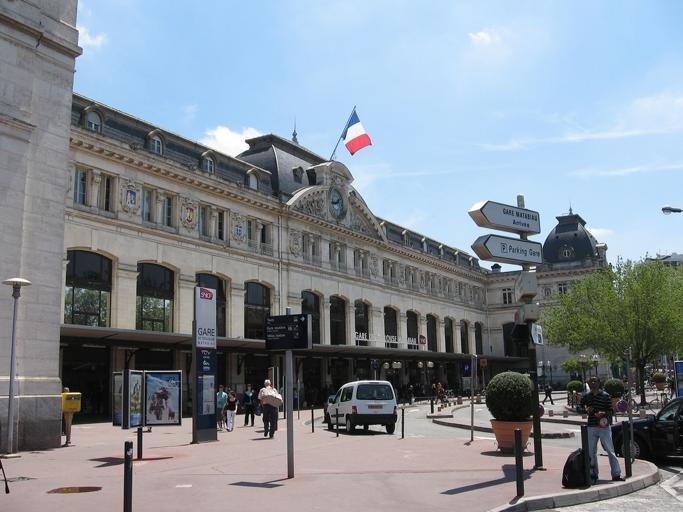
259 387 284 407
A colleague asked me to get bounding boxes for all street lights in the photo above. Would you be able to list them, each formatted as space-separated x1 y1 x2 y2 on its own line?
547 359 552 387
2 277 32 453
591 354 599 377
417 359 435 399
384 360 401 389
578 354 587 390
537 360 545 388
661 206 683 215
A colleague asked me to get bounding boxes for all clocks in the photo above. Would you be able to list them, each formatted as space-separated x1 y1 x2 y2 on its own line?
326 184 348 220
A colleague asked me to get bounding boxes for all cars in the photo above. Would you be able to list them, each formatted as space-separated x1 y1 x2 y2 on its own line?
601 396 683 459
323 394 349 422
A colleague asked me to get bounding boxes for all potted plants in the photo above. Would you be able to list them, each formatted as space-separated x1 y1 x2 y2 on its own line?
600 378 625 411
566 380 586 407
484 369 538 454
652 373 667 392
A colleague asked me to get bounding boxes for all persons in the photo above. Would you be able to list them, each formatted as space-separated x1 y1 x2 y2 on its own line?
542 382 554 405
431 382 435 394
216 378 283 438
435 382 444 405
576 377 625 484
408 385 413 399
668 376 676 399
62 387 69 436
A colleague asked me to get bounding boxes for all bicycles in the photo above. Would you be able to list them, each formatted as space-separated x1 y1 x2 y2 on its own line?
623 393 637 414
649 390 672 414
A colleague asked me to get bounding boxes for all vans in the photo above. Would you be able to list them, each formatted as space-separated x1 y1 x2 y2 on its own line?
327 379 397 434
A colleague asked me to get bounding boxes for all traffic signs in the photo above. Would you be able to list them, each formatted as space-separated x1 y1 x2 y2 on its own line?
514 305 526 325
480 201 540 233
514 275 524 297
486 235 541 263
531 323 545 344
264 315 308 349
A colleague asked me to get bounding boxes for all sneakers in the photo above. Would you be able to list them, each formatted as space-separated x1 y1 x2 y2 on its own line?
269 431 274 438
264 429 268 436
612 475 625 481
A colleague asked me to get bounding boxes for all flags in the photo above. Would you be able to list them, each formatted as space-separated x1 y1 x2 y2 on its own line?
340 110 372 156
